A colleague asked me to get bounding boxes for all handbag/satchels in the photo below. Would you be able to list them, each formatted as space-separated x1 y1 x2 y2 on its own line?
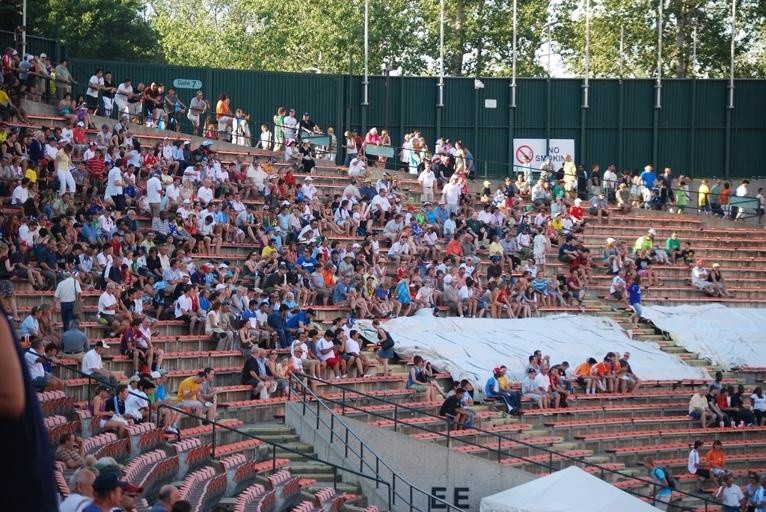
73 301 83 319
378 328 394 349
654 467 676 488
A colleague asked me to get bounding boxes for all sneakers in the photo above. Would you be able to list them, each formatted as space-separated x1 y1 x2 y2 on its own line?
165 424 179 434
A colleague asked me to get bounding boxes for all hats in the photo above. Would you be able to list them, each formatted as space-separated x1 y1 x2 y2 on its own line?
117 479 143 493
493 368 504 374
95 340 111 349
648 228 657 236
606 238 616 245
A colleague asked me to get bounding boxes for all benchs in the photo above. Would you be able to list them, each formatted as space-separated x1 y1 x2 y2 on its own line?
0 102 766 512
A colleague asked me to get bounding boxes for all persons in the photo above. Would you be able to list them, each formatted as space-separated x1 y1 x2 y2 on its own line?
2 48 766 512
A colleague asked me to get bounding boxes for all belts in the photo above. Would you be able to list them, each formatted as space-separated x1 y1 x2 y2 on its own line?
64 351 82 354
